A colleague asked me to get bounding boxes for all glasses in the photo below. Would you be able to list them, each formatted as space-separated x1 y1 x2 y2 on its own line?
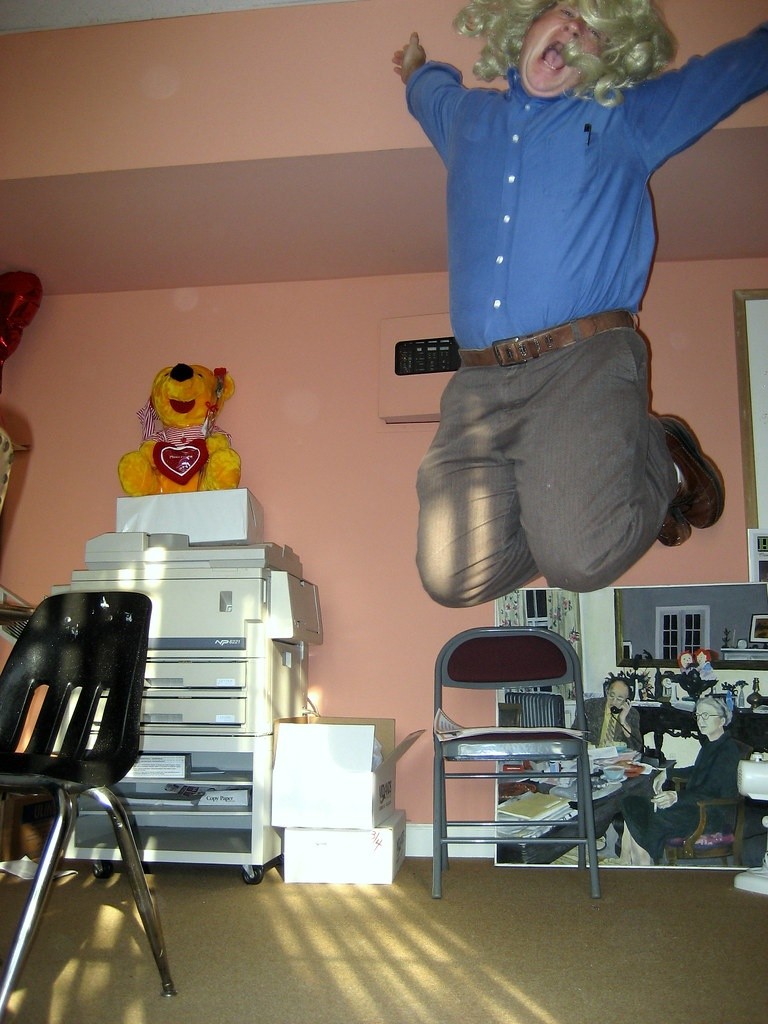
691 712 719 721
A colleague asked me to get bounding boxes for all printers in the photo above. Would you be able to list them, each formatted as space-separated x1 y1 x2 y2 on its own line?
53 526 324 866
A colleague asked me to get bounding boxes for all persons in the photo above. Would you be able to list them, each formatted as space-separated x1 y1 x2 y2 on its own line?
393 0 768 609
678 649 716 682
570 678 643 851
604 697 741 867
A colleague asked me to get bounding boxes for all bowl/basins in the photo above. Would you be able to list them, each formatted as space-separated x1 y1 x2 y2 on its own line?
624 764 646 778
603 766 624 781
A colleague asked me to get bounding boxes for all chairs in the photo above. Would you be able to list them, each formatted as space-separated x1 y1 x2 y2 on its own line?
661 737 755 869
0 590 178 1024
433 626 603 901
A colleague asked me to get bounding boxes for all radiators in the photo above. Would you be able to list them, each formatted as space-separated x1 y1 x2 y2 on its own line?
505 691 567 729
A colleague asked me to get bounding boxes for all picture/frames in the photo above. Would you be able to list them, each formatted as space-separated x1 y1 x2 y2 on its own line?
748 613 768 644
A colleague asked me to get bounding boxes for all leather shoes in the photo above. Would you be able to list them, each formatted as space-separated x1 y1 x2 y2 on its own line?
656 416 723 529
657 506 692 547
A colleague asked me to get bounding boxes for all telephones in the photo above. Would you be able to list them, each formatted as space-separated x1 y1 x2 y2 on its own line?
610 698 631 714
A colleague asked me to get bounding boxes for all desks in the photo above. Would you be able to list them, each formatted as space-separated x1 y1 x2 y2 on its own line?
720 647 768 661
496 759 678 864
725 708 768 754
629 697 737 752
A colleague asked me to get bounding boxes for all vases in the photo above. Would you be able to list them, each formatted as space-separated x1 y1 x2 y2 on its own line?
728 689 745 712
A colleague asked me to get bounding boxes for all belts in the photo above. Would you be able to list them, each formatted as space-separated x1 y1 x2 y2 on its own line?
457 310 634 368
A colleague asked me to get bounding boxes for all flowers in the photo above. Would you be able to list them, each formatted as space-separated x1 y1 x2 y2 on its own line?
601 666 656 702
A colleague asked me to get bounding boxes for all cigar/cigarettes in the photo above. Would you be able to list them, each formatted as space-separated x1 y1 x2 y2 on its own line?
654 803 657 813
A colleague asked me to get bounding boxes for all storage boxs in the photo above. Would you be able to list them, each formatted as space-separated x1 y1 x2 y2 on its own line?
114 488 266 548
271 717 426 885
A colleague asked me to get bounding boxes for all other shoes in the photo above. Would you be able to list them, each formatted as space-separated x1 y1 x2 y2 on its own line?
596 836 606 851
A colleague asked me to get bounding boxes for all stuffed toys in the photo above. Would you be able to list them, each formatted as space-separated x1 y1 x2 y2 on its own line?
118 362 241 497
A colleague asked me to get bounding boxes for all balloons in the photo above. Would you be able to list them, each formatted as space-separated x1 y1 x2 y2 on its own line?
0 271 43 396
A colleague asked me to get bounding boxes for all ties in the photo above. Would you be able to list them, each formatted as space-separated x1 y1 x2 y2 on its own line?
603 715 616 745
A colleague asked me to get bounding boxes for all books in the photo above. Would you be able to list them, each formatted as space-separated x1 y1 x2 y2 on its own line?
497 792 579 839
